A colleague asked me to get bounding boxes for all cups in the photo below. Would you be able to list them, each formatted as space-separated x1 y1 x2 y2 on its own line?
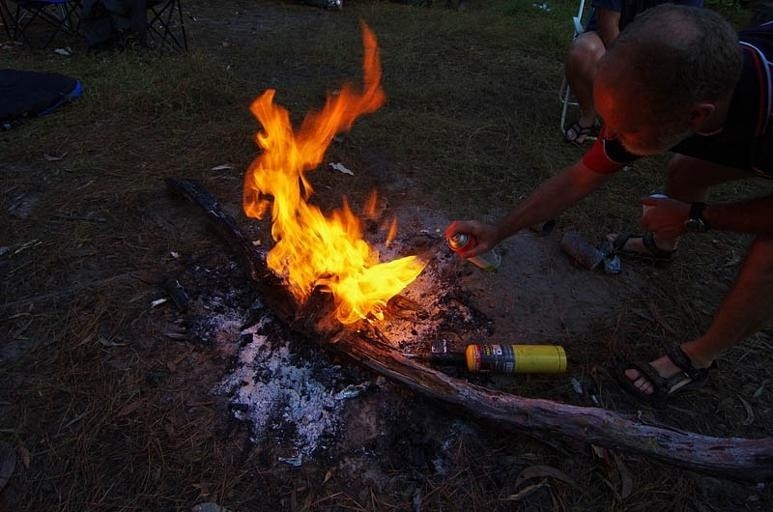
530 217 555 236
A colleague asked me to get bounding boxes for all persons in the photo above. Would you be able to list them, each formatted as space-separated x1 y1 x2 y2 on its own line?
445 3 773 396
563 2 647 145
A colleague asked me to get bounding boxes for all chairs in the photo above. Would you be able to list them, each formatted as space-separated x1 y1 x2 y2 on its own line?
556 2 604 146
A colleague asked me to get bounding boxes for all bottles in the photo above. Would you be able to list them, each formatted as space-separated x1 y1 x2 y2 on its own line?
557 233 603 271
426 343 567 373
444 231 502 274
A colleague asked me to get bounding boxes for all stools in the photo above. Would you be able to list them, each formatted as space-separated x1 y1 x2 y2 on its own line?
93 1 190 58
0 1 87 54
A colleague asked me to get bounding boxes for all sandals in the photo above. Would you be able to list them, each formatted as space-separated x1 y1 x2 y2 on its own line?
617 341 711 402
566 119 599 145
604 230 680 263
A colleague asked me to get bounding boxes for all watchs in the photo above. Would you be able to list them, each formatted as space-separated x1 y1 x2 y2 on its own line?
684 200 707 235
